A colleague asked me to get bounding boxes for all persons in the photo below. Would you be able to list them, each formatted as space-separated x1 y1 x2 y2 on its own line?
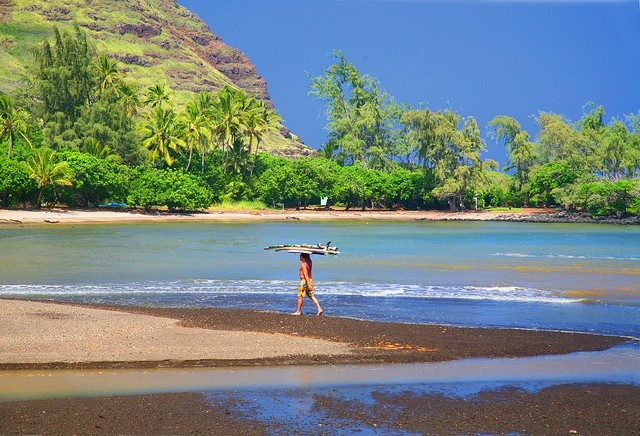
539 200 544 207
524 199 528 208
290 253 323 315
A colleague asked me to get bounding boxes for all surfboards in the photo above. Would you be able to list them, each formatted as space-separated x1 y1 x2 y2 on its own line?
264 241 340 256
